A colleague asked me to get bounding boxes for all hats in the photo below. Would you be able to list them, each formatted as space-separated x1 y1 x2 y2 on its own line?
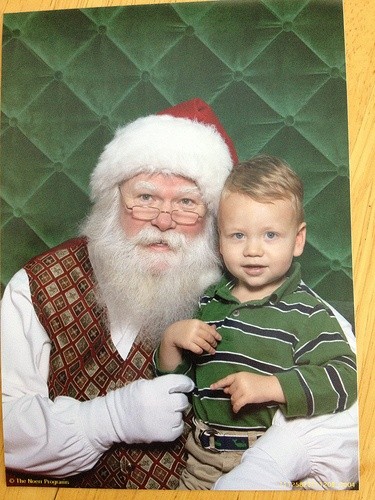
87 96 239 211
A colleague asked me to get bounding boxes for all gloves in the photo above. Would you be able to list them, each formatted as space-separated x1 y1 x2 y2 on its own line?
105 372 195 444
211 452 293 490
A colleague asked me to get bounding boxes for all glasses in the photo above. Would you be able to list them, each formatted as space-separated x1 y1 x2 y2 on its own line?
118 186 209 226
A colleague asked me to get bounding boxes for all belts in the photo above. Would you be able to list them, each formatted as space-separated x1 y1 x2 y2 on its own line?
191 415 263 452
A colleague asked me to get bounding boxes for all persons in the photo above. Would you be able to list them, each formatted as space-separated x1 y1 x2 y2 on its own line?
149 152 358 493
0 97 360 494
